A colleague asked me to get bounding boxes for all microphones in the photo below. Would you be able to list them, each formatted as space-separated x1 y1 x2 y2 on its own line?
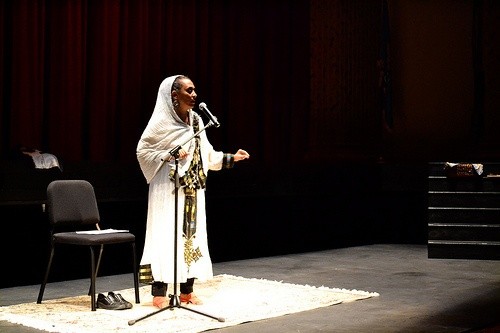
199 102 220 128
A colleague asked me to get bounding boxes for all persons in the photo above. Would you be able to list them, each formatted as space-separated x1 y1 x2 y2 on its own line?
136 74 250 310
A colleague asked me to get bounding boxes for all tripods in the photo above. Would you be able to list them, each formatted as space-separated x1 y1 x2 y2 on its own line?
128 119 224 326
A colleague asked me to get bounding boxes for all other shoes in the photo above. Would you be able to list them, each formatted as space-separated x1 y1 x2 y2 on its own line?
180 292 204 305
153 296 170 308
97 292 133 310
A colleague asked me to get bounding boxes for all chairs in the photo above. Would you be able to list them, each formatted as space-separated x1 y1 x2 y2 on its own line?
37 180 140 311
